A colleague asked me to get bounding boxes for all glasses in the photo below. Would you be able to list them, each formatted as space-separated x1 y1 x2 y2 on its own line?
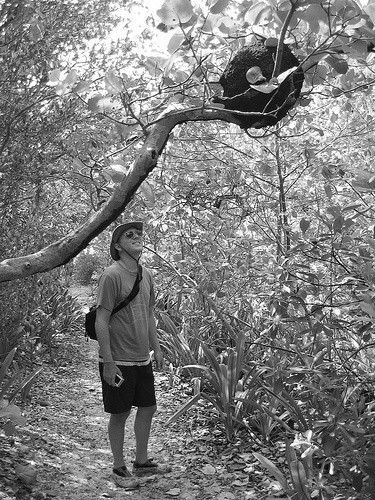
121 230 142 239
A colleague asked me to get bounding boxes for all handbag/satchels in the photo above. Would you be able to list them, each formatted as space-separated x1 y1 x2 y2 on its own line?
84 304 99 342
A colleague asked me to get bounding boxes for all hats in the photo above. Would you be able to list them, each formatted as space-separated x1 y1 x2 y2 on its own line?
110 221 143 261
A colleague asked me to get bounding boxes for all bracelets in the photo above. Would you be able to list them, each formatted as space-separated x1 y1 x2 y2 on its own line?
104 361 115 364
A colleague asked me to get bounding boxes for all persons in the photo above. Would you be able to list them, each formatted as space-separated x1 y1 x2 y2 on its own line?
93 221 172 488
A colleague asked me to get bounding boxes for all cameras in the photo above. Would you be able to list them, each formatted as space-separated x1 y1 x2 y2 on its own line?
115 374 124 388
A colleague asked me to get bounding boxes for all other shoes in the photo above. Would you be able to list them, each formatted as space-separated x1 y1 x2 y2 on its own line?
130 459 171 473
112 466 140 487
